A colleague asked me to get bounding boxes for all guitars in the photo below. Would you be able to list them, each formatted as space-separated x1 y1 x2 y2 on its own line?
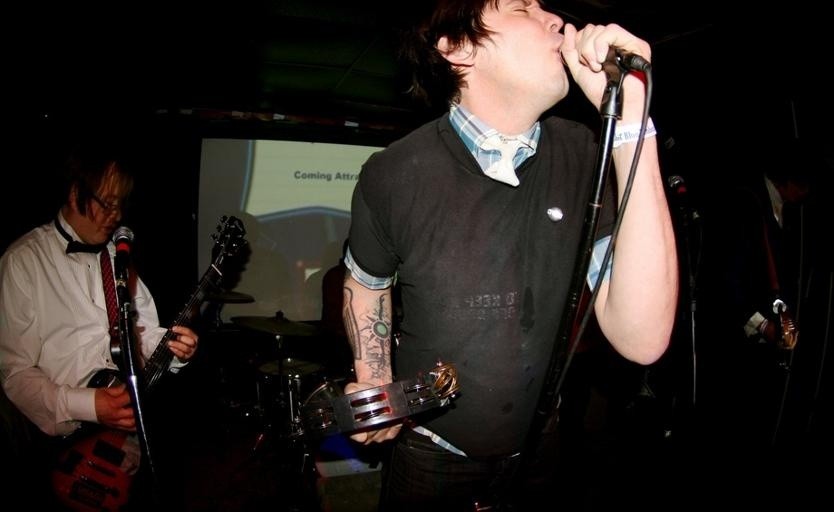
41 216 245 511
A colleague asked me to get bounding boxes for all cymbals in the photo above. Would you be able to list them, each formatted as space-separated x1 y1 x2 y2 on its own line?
251 340 326 376
229 315 326 338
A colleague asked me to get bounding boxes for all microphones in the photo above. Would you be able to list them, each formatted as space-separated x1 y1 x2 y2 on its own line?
559 39 650 70
668 175 699 220
113 224 133 303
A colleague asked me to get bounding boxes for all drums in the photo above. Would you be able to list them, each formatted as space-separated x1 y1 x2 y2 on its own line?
304 379 355 429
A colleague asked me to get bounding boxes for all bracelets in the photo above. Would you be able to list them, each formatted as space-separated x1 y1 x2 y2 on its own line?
611 114 659 149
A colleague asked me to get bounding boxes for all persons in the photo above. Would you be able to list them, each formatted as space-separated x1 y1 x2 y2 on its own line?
0 150 205 510
693 138 823 511
317 236 352 377
329 0 689 510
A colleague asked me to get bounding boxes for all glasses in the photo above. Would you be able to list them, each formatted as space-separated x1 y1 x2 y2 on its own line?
91 193 124 217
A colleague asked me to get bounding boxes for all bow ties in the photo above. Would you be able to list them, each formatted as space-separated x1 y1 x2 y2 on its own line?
53 217 106 255
